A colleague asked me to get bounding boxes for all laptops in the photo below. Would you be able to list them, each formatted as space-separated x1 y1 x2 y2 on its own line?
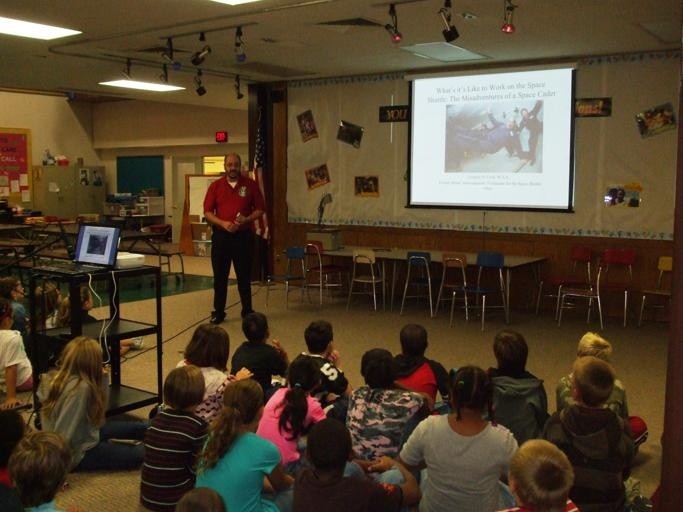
31 221 121 276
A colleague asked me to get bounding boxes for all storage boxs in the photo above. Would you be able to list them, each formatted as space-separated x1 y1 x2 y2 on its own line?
305 228 343 250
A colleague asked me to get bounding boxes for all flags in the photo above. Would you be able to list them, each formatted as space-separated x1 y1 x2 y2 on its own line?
253 116 271 243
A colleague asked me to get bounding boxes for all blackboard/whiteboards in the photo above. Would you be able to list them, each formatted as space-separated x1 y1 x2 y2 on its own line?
185 174 222 224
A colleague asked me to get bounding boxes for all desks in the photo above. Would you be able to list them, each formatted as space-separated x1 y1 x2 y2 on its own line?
304 241 548 310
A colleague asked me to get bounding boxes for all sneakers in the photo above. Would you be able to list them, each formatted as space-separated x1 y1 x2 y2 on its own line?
131 336 142 349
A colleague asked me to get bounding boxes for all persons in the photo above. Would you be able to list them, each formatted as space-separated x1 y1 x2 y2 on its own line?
515 100 542 164
448 109 528 159
614 189 626 205
139 313 649 512
202 154 265 326
1 276 149 511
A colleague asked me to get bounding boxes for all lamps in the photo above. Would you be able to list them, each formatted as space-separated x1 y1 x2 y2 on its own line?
372 1 520 42
161 21 261 70
104 56 260 100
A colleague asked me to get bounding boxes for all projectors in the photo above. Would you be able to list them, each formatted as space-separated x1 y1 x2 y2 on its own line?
115 251 145 269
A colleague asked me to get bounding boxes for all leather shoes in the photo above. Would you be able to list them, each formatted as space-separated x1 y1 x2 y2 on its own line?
210 315 220 325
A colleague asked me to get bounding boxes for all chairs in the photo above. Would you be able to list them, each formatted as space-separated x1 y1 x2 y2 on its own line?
304 240 351 306
433 251 470 319
447 251 507 331
346 249 389 311
264 246 308 310
399 251 440 318
555 262 605 329
588 246 637 326
637 255 671 331
1 214 185 331
535 244 597 319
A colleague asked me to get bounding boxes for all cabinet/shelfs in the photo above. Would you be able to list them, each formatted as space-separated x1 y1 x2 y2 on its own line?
32 166 75 218
23 260 163 425
76 165 108 217
